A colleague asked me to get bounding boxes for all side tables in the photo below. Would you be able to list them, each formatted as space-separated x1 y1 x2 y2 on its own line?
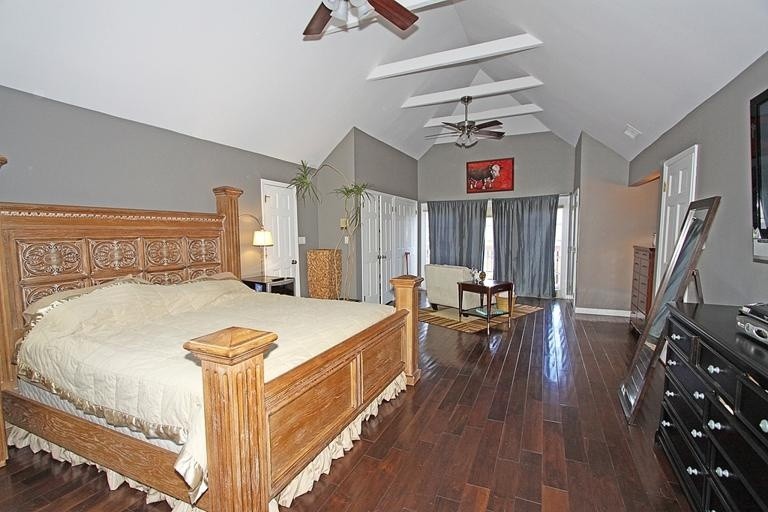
457 280 513 336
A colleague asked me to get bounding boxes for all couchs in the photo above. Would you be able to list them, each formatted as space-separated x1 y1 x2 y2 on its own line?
425 264 496 317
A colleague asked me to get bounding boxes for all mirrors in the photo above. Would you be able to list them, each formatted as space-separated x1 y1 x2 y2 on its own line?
617 196 721 425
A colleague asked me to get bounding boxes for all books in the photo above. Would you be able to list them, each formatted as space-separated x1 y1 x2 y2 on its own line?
476 308 504 316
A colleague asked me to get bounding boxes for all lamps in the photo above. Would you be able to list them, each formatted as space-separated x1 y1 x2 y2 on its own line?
239 215 274 258
322 0 378 23
456 134 478 147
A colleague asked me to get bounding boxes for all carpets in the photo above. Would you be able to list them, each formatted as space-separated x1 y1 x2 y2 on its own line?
419 303 544 334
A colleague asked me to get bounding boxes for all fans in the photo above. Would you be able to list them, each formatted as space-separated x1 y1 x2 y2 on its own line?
424 97 505 140
303 0 419 36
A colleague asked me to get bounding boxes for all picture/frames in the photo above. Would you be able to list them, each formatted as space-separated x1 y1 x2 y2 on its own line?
466 158 514 194
750 89 768 240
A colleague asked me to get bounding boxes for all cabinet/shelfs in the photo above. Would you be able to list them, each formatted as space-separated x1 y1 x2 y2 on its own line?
654 302 768 512
629 245 655 336
360 188 419 305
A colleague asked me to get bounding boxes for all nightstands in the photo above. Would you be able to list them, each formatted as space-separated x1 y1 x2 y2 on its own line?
242 276 296 296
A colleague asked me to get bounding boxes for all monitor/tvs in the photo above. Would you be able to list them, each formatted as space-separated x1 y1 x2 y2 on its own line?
749 87 768 264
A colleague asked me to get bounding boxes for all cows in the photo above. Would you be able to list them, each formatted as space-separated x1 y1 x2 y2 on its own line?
468 164 503 190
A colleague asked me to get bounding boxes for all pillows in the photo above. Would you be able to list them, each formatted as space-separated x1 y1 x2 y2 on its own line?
22 274 148 329
180 271 238 296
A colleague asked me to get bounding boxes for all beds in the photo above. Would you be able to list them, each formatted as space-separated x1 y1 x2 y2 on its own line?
0 157 424 511
